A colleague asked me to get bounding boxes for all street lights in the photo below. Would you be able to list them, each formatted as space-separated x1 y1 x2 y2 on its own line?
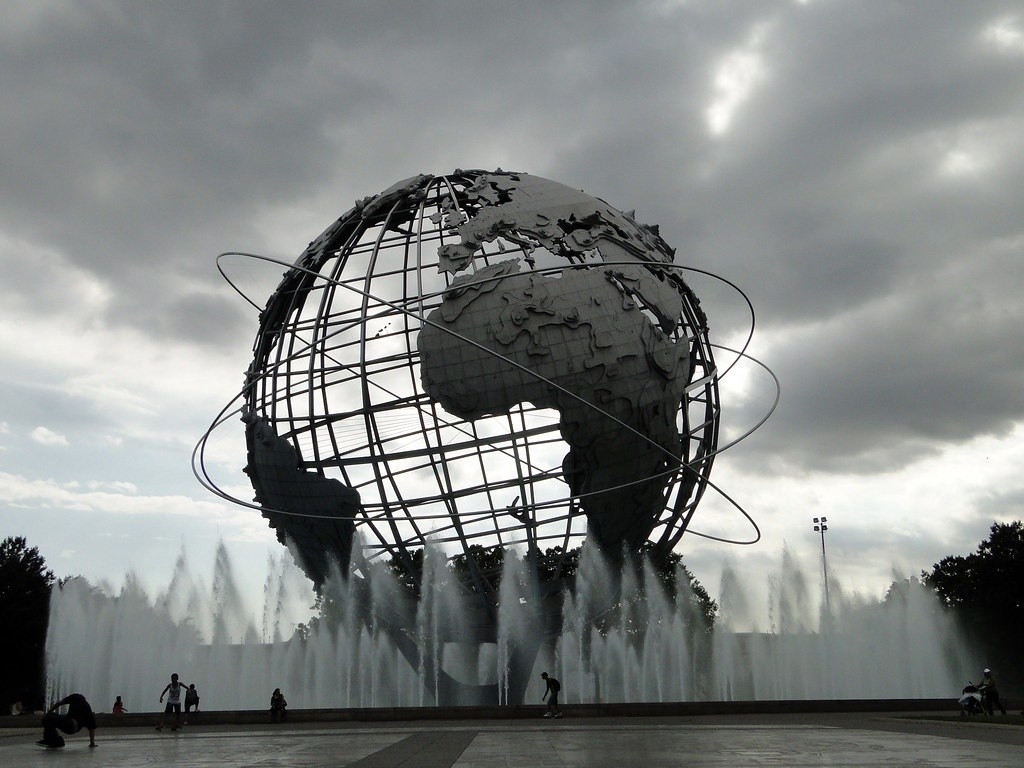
813 517 829 614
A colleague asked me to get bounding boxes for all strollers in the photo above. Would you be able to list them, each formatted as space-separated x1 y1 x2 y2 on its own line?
957 679 991 719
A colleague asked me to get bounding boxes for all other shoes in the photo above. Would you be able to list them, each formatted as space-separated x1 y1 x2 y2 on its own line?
36 737 55 746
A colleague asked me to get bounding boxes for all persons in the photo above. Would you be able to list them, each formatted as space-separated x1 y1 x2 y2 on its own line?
36 693 98 748
12 701 25 716
113 696 128 713
974 668 1006 716
540 672 562 719
155 674 200 732
266 688 288 723
